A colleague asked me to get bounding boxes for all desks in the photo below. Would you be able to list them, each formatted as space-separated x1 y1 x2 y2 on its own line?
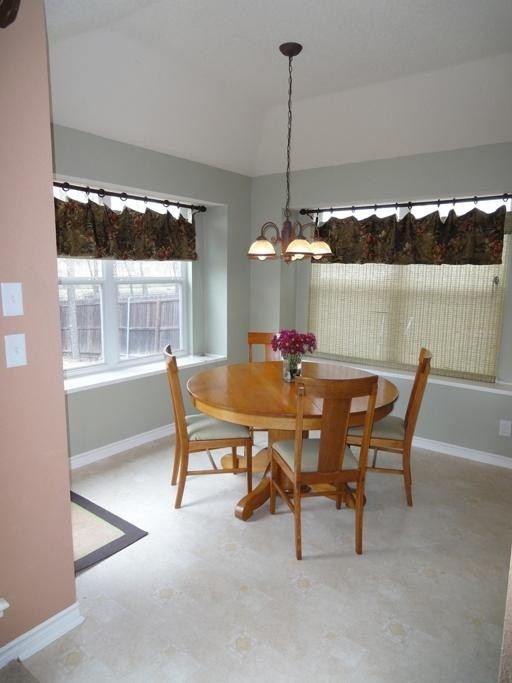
187 360 400 522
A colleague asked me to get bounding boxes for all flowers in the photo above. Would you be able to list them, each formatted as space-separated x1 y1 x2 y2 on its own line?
270 328 318 356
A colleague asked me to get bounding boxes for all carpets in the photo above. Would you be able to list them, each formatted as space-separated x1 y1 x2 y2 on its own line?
69 490 149 577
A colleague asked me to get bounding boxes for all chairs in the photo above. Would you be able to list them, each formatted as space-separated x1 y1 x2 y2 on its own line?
163 343 253 509
345 346 433 506
248 332 310 446
270 376 379 560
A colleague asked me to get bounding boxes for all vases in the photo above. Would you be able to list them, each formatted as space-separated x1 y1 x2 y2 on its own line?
283 352 303 383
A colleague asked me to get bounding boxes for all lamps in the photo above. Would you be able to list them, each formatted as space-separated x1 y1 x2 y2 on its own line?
243 38 333 268
